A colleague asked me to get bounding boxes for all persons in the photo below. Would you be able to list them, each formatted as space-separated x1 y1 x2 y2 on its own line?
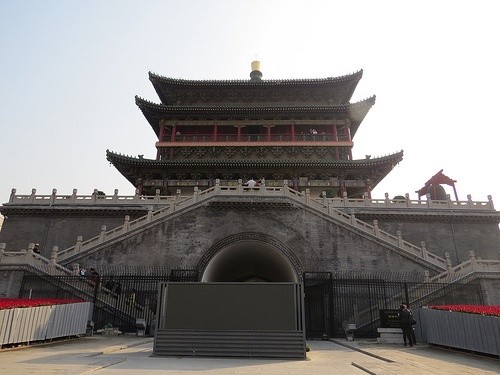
399 303 418 347
33 244 41 260
128 289 136 308
78 267 87 282
244 177 264 190
309 128 317 134
427 181 447 204
106 277 121 300
90 268 100 288
